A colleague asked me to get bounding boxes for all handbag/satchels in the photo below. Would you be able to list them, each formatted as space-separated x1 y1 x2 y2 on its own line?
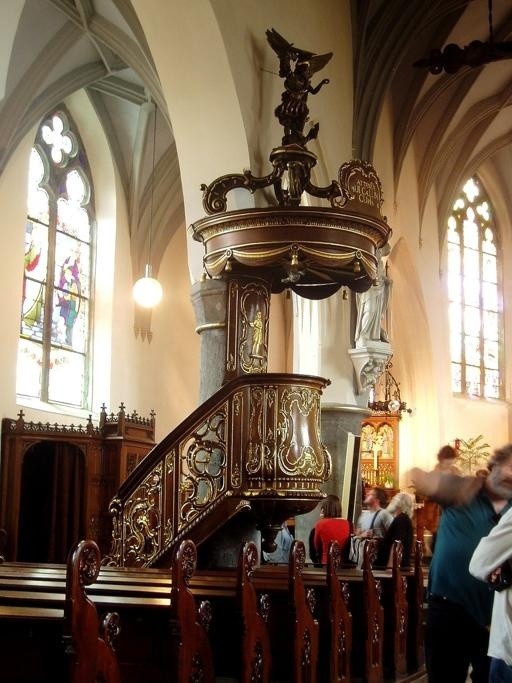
348 533 404 570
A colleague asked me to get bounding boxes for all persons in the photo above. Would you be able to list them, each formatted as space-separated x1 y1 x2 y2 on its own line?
260 517 298 565
422 443 463 554
409 444 511 682
467 503 512 682
247 310 266 360
309 492 355 567
385 470 422 524
353 484 395 567
307 503 329 568
353 242 395 349
372 491 417 571
273 44 331 145
